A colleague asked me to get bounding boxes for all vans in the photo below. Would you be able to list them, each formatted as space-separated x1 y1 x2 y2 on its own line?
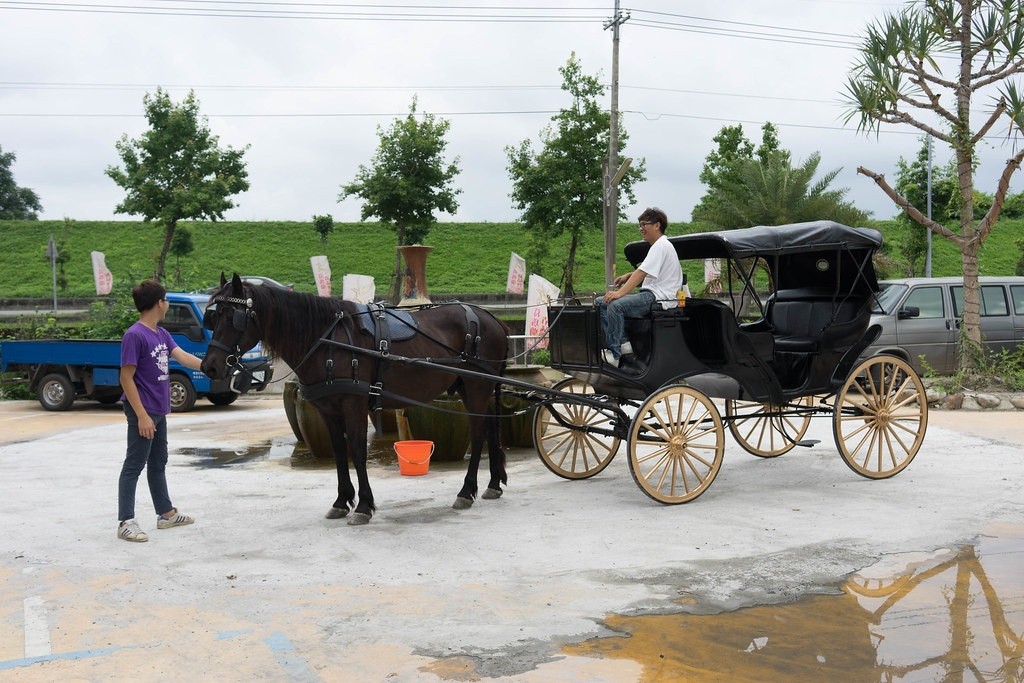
847 275 1024 394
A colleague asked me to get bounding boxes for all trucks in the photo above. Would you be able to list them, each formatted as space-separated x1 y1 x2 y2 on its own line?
0 292 274 413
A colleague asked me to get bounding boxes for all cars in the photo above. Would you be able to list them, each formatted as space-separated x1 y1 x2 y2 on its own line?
206 276 295 294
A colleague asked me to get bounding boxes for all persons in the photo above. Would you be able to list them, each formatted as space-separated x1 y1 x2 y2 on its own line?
594 207 684 367
118 280 203 542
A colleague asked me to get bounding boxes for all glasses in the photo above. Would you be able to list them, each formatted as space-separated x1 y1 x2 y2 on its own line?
162 299 170 304
638 222 657 228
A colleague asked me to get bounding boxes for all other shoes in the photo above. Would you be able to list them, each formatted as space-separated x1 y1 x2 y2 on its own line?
600 348 619 367
620 342 634 354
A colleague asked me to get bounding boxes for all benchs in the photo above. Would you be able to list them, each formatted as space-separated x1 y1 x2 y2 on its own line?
603 273 870 369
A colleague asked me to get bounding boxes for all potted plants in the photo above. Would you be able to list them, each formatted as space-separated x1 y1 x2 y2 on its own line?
530 348 566 383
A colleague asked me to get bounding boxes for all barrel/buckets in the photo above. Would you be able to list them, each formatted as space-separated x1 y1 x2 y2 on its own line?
283 364 552 462
393 441 435 475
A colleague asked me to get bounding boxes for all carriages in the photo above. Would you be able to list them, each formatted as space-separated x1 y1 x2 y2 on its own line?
200 219 928 527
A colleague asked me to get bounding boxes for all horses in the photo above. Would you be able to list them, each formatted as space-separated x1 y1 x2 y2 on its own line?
199 268 513 526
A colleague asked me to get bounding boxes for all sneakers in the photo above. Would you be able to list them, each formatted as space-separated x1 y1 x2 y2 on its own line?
117 519 147 541
158 506 194 529
